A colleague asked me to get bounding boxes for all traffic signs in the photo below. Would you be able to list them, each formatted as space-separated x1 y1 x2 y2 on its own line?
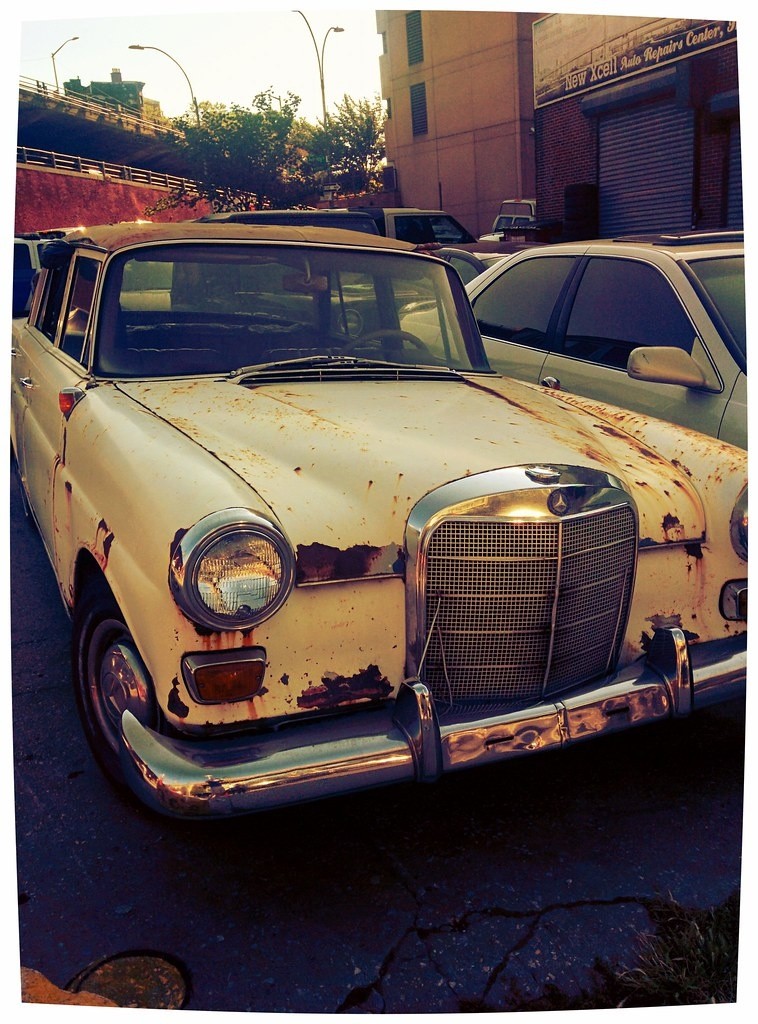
318 182 343 193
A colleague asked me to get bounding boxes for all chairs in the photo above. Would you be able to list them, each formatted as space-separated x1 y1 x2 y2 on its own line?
258 347 382 364
124 347 230 375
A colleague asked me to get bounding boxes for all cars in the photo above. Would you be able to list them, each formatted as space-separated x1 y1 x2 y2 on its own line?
12 198 748 809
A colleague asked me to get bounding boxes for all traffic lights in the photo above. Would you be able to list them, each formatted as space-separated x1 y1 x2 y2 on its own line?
326 161 347 172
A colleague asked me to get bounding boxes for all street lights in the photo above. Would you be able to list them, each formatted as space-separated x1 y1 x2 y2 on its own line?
51 36 81 94
291 9 345 130
127 44 201 128
